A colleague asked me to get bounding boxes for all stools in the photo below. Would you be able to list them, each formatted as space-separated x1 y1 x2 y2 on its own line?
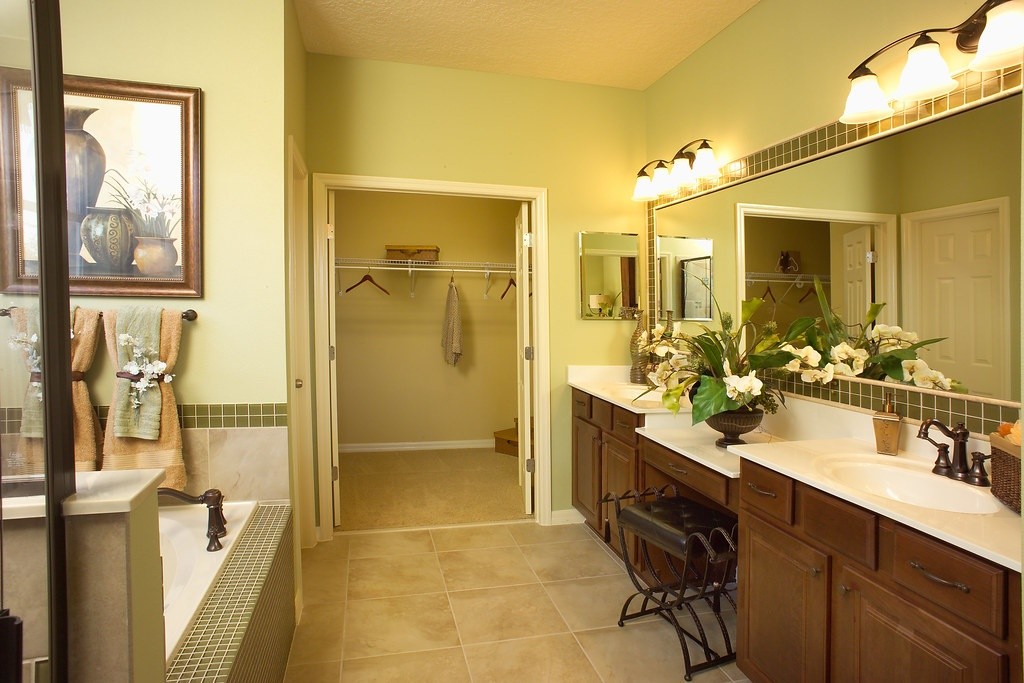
593 482 740 681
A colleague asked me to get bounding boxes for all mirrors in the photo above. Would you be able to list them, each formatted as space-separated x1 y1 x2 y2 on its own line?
579 230 640 320
656 235 716 321
646 47 1024 441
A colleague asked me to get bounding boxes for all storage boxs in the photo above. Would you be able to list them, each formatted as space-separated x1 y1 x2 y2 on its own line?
494 428 534 458
385 245 440 260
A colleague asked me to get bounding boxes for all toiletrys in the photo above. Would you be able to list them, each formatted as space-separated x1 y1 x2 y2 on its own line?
872 391 903 456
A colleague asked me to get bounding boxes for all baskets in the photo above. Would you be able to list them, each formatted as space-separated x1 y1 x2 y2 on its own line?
989 431 1021 516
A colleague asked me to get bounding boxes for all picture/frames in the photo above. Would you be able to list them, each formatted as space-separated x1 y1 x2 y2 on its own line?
0 65 203 299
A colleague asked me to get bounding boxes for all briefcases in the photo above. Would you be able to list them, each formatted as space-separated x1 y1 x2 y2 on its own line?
385 244 440 262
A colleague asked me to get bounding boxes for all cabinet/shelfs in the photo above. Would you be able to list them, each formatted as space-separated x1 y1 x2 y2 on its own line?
572 387 736 591
736 456 1024 683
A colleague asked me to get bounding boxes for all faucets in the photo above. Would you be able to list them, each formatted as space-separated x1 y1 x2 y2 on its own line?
918 418 991 487
156 487 228 553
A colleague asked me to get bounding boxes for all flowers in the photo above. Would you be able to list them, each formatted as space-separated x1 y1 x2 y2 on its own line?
797 276 963 393
102 168 181 237
116 332 175 410
628 269 835 427
9 328 81 403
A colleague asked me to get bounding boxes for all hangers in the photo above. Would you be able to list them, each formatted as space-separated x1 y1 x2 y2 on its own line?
345 266 390 296
500 269 516 300
450 268 454 282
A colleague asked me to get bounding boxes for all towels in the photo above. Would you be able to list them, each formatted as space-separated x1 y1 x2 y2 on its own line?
9 306 189 494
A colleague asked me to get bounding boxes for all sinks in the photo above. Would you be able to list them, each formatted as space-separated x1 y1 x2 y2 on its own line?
812 449 1003 519
608 381 666 404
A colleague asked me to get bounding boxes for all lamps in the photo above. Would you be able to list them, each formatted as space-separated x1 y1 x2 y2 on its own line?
838 0 1024 125
632 138 720 201
590 294 612 316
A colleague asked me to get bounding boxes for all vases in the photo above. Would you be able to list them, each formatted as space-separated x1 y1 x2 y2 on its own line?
81 207 142 271
705 409 764 448
630 310 650 384
64 105 106 267
134 237 178 277
661 310 680 343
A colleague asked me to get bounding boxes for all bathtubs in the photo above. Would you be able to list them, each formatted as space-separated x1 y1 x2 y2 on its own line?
158 513 196 615
